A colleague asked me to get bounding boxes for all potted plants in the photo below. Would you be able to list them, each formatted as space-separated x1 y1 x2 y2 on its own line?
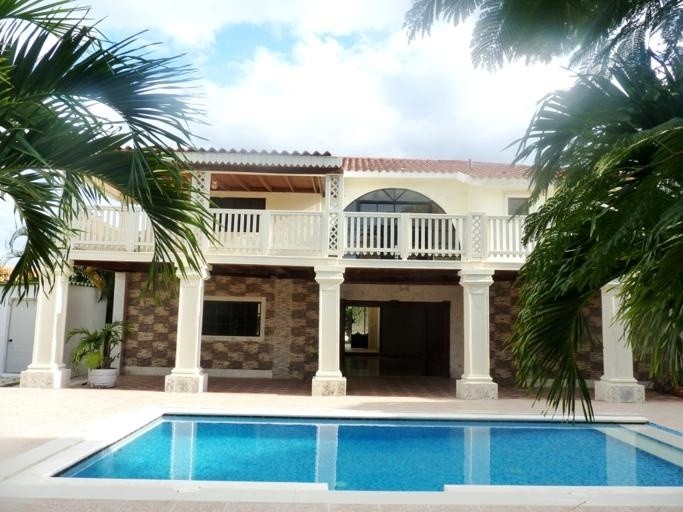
66 320 134 387
